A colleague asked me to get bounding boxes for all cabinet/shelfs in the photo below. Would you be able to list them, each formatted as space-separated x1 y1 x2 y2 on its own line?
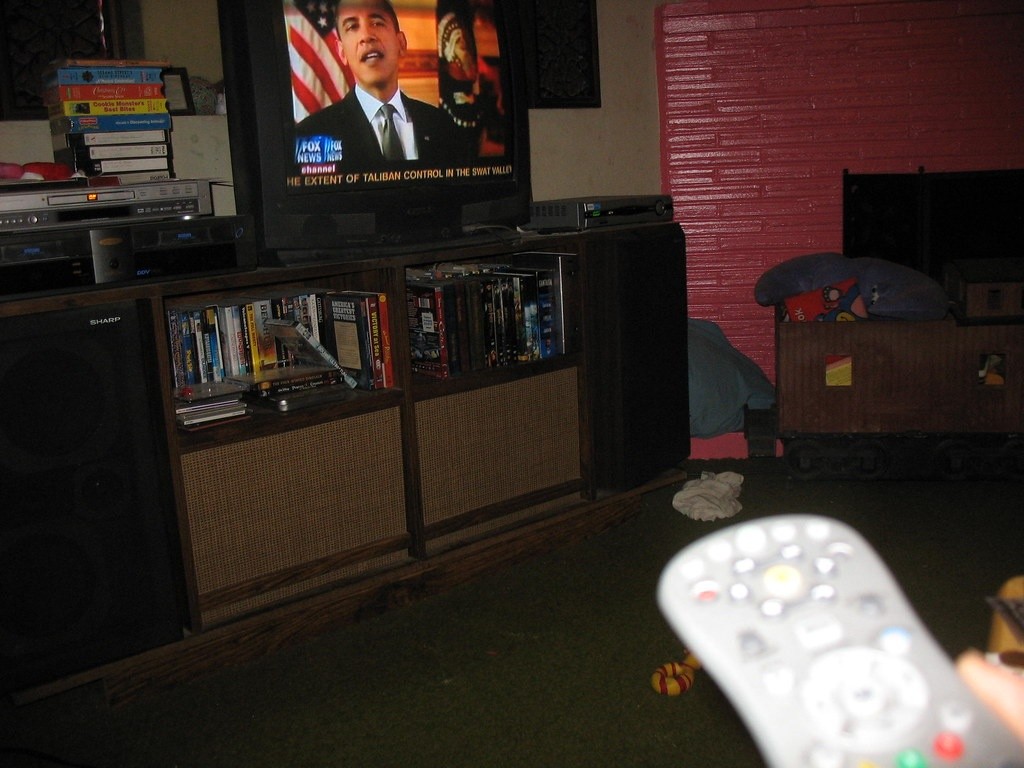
0 221 694 716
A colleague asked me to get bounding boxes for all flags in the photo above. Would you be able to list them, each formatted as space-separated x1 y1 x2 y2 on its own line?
437 0 482 141
282 0 356 124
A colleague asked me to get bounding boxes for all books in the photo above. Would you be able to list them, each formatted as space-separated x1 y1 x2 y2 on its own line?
42 59 175 188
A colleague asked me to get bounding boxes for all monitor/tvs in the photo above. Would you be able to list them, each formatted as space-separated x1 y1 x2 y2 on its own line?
219 0 533 268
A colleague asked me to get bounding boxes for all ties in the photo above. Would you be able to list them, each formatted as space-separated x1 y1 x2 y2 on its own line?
380 104 406 164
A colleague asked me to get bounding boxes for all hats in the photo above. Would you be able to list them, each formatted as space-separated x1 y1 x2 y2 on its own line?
439 12 461 62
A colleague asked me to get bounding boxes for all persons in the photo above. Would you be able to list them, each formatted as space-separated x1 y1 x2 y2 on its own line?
294 1 461 175
